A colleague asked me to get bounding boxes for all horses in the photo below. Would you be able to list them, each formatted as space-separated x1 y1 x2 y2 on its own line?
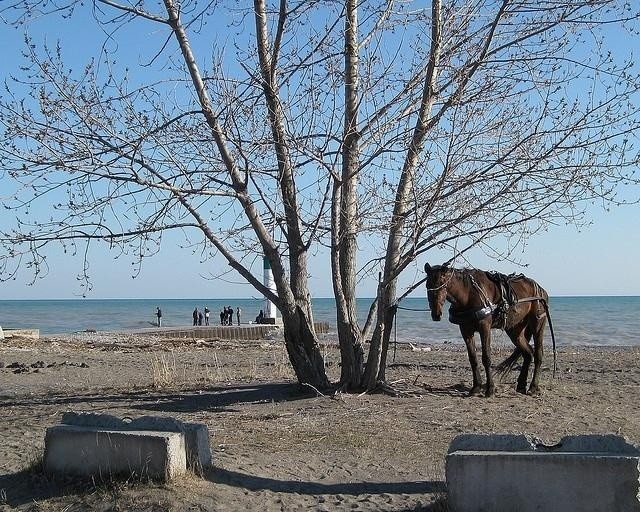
424 262 549 399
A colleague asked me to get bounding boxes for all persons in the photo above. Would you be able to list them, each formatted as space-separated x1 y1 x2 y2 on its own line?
237 306 241 326
220 305 233 326
254 310 263 324
203 306 210 326
193 307 203 326
155 307 162 328
